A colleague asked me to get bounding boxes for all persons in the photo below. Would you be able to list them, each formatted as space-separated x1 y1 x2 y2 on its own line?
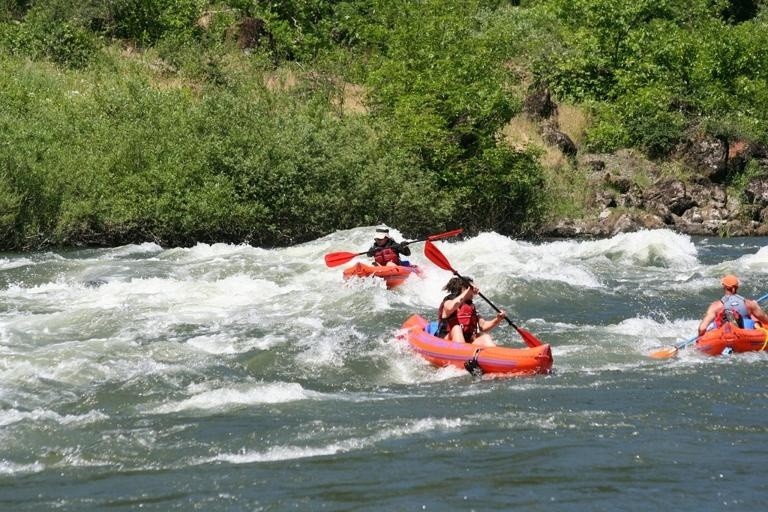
697 274 768 337
435 274 512 346
366 224 412 267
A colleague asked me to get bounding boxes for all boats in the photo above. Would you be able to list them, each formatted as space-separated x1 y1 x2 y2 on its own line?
343 261 424 290
693 321 768 357
399 313 553 377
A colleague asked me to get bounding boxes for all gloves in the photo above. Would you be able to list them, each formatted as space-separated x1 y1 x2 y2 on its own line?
400 241 408 249
368 248 377 256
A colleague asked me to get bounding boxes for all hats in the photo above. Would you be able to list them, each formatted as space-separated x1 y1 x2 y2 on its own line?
720 274 738 288
373 226 389 240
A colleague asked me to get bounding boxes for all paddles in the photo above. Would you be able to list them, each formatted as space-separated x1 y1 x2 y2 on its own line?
650 294 768 359
423 238 542 347
324 229 464 268
